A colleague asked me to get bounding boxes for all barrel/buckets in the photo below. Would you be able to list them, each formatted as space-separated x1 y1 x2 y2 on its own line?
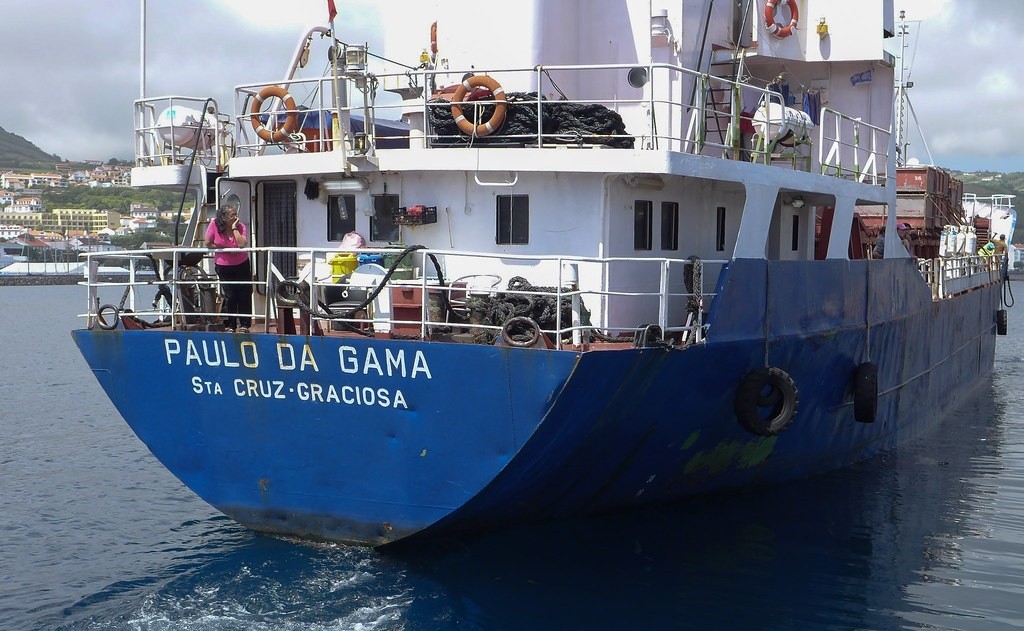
327 244 413 282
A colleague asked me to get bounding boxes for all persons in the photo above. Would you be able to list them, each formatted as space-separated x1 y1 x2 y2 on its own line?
767 72 789 105
871 222 920 259
990 232 1007 263
208 203 252 333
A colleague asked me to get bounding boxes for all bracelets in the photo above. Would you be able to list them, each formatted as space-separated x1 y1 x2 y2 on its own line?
232 228 238 231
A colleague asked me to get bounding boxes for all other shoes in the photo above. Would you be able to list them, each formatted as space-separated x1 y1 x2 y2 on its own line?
228 328 236 333
239 327 249 334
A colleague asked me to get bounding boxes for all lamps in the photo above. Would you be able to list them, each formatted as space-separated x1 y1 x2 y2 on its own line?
782 196 804 209
345 45 368 70
626 174 665 191
321 178 367 193
651 9 668 36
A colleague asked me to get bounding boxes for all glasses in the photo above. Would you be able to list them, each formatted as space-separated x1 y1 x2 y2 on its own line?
226 214 239 220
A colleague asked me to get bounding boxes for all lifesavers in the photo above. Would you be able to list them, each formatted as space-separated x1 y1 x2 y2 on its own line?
996 310 1008 335
853 362 879 424
250 86 296 143
766 0 799 37
450 75 506 136
732 367 799 437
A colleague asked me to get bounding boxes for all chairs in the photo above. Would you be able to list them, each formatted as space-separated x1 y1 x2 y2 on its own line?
323 286 374 330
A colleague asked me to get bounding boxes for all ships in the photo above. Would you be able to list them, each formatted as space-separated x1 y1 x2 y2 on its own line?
74 0 1018 554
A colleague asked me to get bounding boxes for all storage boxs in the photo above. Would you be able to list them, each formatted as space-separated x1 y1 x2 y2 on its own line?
391 205 438 226
814 166 964 259
391 280 468 336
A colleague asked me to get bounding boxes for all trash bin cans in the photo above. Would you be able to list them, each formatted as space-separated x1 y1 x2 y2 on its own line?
330 243 414 284
